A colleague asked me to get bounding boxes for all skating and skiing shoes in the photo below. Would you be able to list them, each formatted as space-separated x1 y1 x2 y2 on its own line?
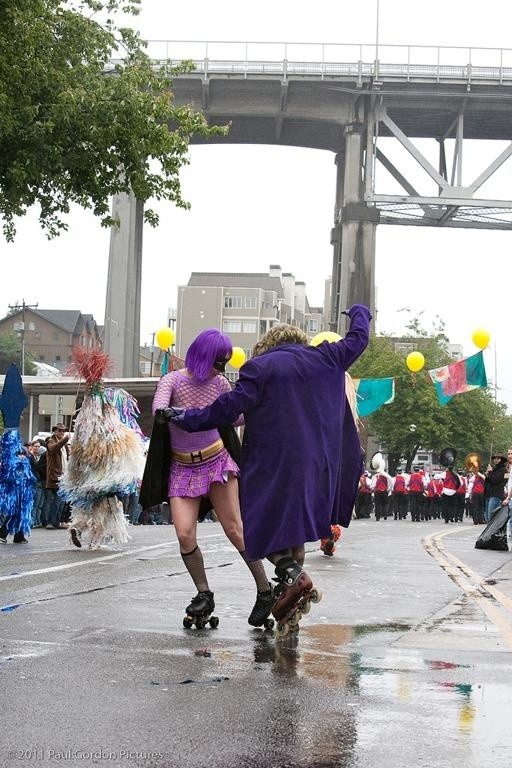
248 581 280 630
270 565 323 637
319 523 342 557
183 590 219 630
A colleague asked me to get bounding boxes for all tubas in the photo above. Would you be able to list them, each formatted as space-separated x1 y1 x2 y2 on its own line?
465 453 486 480
371 451 394 490
440 447 460 489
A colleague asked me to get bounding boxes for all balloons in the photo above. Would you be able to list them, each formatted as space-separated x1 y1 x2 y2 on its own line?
229 347 245 368
471 327 491 349
406 351 425 371
156 328 175 353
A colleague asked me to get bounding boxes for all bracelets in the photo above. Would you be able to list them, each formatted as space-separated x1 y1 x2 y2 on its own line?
507 497 510 500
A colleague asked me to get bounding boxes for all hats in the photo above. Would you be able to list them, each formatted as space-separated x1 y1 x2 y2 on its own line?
491 451 508 463
52 423 66 432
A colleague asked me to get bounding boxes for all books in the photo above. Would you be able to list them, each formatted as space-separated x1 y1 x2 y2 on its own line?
311 331 343 347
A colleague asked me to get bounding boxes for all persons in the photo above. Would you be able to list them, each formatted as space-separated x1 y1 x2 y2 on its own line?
501 445 512 551
484 451 507 524
152 328 276 626
351 466 486 525
0 387 172 551
170 304 372 622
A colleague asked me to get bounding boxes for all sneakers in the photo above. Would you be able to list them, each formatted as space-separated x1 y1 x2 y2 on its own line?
31 521 72 529
0 528 8 544
354 512 487 525
13 534 29 544
70 527 82 548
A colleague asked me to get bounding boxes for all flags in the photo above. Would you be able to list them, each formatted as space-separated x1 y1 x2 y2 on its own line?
353 377 396 417
428 351 488 408
